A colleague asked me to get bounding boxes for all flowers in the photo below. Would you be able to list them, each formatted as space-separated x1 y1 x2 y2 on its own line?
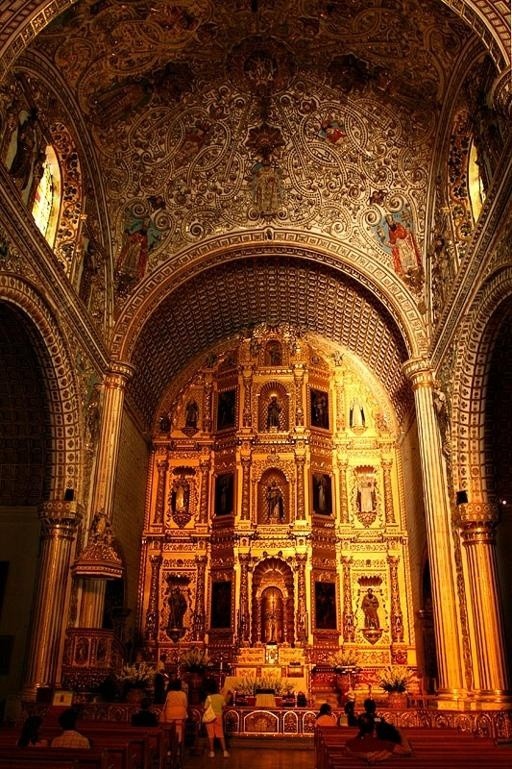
184 650 209 672
328 650 364 672
378 666 419 692
116 662 156 685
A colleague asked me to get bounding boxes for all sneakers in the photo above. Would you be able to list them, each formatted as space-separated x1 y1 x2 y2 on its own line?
223 751 231 757
208 752 214 757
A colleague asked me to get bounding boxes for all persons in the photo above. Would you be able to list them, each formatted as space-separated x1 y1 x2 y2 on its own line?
344 720 413 762
344 713 394 756
265 396 284 430
361 587 381 629
113 215 149 297
338 701 358 727
49 709 92 750
184 396 198 430
358 474 377 513
167 584 188 637
15 715 49 748
266 479 284 522
360 697 382 722
245 122 286 223
159 678 189 759
383 213 425 296
198 677 231 758
313 703 338 726
172 478 187 515
85 670 181 705
307 391 328 427
130 698 158 728
350 393 364 428
316 470 329 513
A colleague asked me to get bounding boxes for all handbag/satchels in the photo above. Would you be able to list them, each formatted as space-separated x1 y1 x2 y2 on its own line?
202 704 216 723
160 712 165 722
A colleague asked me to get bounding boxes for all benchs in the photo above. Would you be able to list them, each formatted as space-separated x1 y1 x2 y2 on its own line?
314 725 512 769
0 721 198 769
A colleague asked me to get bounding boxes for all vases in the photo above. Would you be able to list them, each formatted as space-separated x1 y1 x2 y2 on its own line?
386 692 408 709
123 685 144 704
336 666 354 704
190 672 203 704
